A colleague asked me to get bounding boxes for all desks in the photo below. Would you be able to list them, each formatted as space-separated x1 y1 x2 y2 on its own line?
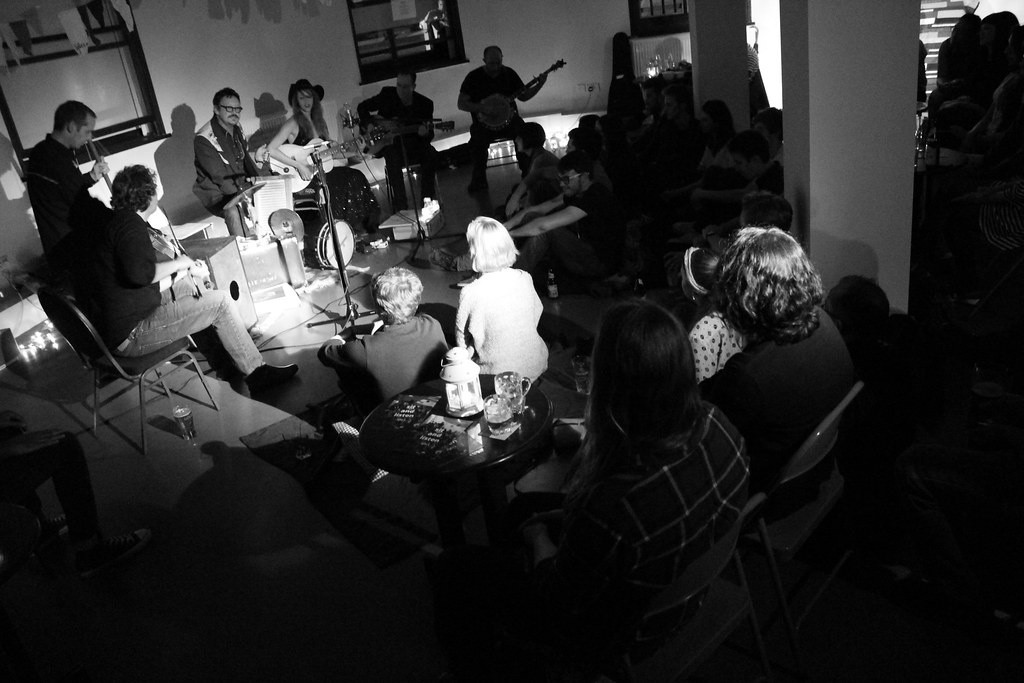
358 373 552 549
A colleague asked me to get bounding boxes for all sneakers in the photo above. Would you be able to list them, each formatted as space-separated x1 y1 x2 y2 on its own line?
94 528 151 568
52 514 68 535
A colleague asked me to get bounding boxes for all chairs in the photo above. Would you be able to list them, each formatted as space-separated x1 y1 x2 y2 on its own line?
147 203 211 239
738 381 869 676
37 285 220 456
501 493 774 683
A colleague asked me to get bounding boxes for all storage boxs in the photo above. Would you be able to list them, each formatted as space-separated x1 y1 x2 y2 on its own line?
378 208 444 241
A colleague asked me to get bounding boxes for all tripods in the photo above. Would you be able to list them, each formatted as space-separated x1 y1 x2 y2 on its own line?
308 115 467 330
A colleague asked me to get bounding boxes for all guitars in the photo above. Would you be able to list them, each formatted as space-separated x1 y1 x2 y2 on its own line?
268 124 390 194
358 114 456 159
476 58 568 131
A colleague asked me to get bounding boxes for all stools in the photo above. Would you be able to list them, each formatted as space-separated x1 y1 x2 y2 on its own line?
384 164 442 209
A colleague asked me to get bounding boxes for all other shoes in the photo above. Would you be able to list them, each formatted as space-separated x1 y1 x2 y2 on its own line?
457 276 475 287
429 248 456 271
244 362 299 394
468 182 488 191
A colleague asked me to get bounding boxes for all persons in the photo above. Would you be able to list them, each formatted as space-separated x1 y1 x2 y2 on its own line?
433 295 750 650
317 266 449 409
547 245 744 392
458 46 546 193
427 77 793 298
266 79 388 244
86 166 298 398
27 100 108 304
192 87 269 237
822 12 1024 614
356 69 441 211
695 227 852 496
455 215 549 382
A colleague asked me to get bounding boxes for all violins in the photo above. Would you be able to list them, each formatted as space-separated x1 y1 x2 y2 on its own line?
147 226 210 278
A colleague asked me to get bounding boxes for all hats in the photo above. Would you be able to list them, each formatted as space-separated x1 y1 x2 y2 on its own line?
288 79 324 106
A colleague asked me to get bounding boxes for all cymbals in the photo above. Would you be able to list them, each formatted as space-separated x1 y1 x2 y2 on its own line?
222 181 267 212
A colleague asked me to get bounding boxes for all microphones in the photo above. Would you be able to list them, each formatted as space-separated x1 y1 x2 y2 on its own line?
346 109 353 135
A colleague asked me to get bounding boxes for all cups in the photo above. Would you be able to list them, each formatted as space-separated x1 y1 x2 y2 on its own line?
172 404 198 441
571 354 592 395
484 395 514 436
494 371 532 413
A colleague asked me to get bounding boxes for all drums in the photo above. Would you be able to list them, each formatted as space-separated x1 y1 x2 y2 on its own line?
302 216 357 270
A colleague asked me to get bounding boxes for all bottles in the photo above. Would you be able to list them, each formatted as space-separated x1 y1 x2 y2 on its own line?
548 269 558 298
423 197 432 208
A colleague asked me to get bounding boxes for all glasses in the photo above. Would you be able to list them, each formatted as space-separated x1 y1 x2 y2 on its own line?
556 173 584 184
220 105 242 113
